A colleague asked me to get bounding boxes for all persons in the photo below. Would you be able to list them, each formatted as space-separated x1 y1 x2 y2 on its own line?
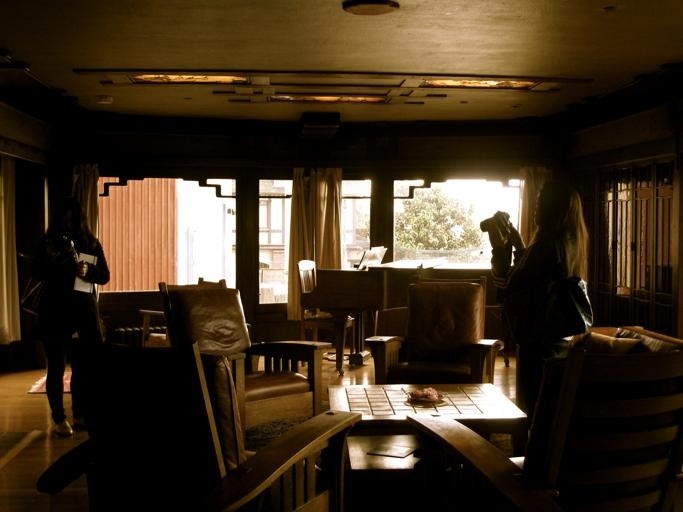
31 191 110 435
485 178 591 454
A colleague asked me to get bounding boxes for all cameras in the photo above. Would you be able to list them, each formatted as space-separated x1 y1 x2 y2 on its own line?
481 213 511 238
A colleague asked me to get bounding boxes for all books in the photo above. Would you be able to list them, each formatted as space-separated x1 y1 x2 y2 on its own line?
73 252 97 293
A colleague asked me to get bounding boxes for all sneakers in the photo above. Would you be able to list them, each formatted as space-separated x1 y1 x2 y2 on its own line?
55 420 72 435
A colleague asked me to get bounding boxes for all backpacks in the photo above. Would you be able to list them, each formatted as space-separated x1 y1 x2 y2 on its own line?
543 278 593 359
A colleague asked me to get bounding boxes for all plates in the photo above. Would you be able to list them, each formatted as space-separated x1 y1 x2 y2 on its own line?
407 392 448 401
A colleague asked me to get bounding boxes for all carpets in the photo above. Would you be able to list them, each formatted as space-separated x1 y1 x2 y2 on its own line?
29 371 73 393
